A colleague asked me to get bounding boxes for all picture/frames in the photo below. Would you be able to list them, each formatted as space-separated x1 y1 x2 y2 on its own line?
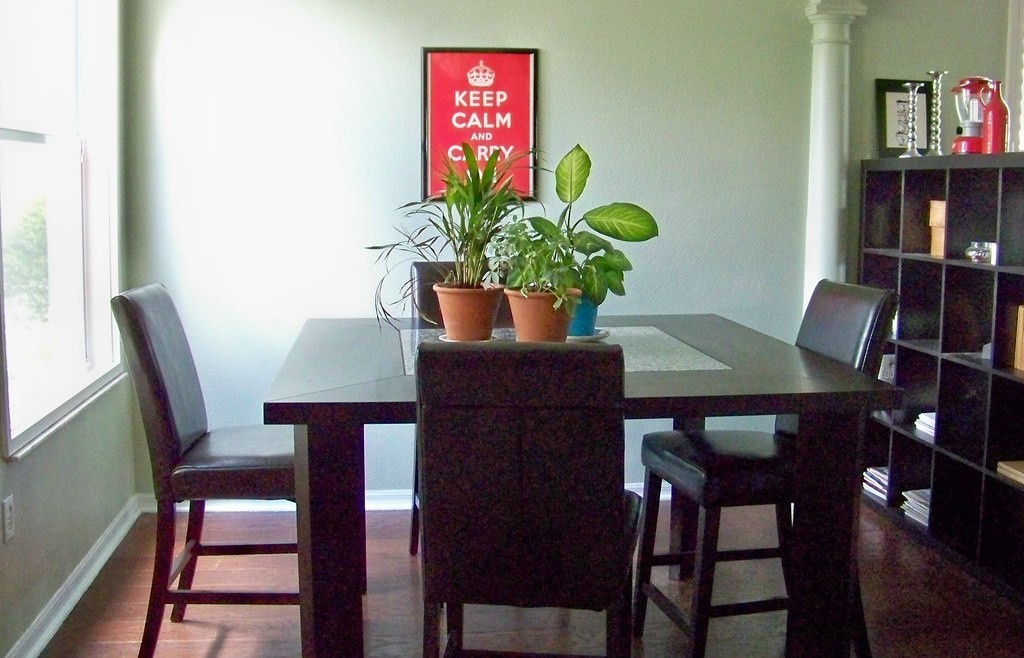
875 78 933 158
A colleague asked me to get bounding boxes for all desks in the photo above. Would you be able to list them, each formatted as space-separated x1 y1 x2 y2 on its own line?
263 313 908 658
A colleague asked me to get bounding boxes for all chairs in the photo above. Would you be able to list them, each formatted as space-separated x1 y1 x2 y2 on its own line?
632 279 900 658
110 281 366 658
414 341 645 658
410 262 516 555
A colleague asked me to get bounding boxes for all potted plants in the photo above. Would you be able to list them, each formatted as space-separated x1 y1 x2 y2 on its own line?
365 141 659 343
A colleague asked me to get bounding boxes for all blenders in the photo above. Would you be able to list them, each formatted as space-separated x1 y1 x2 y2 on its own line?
952 76 993 153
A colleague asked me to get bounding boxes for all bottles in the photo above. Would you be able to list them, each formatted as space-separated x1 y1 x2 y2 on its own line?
965 242 991 264
979 80 1010 154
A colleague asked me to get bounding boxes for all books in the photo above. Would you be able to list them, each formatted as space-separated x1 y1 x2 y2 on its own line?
1014 304 1024 371
914 412 936 444
863 466 889 500
900 489 930 527
997 461 1024 484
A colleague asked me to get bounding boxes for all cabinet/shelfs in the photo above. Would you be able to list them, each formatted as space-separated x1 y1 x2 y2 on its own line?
857 152 1024 606
